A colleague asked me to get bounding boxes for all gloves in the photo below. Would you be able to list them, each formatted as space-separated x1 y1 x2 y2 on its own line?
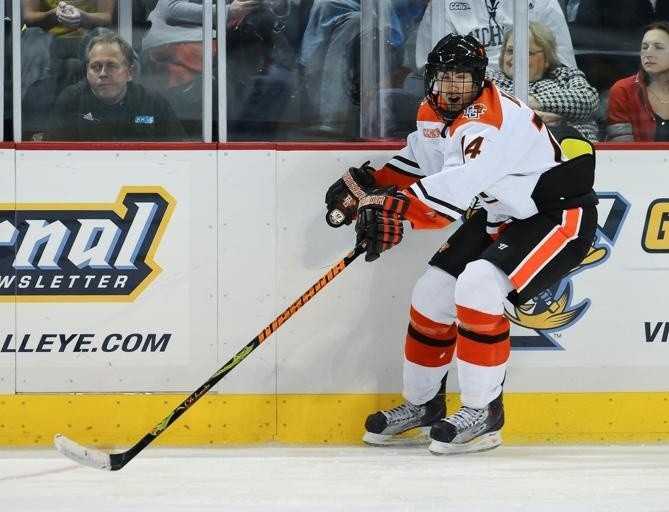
325 160 409 262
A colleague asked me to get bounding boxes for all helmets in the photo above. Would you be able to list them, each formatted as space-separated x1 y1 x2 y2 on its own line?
424 32 489 122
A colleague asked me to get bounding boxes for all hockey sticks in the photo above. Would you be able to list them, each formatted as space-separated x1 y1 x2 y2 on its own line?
54 239 367 472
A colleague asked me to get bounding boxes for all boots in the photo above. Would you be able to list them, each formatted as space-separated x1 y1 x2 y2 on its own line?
364 372 505 444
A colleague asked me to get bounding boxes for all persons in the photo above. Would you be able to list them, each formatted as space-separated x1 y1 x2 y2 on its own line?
10 1 669 145
324 31 598 446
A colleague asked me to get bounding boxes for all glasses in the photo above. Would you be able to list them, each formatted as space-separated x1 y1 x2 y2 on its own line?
504 48 544 56
